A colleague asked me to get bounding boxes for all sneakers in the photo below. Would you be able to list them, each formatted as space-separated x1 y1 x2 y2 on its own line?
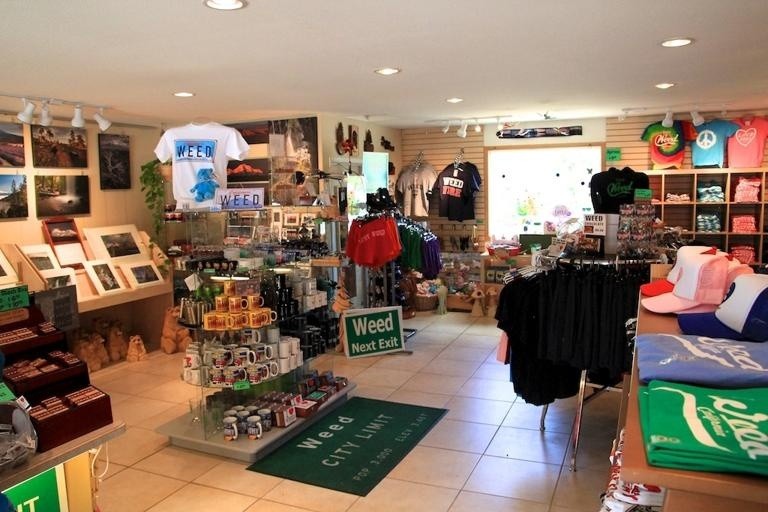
379 187 397 210
366 193 385 212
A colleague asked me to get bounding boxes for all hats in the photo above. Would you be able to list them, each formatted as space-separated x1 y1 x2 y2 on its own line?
640 245 722 297
673 264 754 314
724 255 741 274
677 273 768 343
641 254 729 314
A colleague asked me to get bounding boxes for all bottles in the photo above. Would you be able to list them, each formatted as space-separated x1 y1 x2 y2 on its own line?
178 295 207 325
207 303 212 312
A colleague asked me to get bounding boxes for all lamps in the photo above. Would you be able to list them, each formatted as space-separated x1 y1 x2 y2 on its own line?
441 124 451 134
16 101 35 125
473 118 482 131
456 121 468 138
660 111 673 127
93 112 111 132
690 110 704 126
70 107 84 128
495 116 505 131
41 107 51 125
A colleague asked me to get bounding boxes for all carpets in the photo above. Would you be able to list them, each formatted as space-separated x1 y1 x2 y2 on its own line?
246 395 449 497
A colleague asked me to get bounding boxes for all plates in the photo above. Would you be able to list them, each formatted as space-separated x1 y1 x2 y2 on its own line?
238 257 264 269
224 248 240 259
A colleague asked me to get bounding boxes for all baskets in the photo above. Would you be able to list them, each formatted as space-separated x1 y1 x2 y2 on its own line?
412 293 438 311
438 272 468 289
487 243 521 256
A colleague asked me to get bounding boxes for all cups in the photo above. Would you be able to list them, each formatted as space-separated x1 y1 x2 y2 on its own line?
228 296 248 313
301 305 340 358
281 318 304 337
249 310 268 328
242 312 250 328
280 337 304 374
215 314 234 330
223 280 236 296
269 274 298 321
183 329 247 388
237 411 250 434
246 415 262 440
230 313 249 330
247 296 265 310
223 409 237 417
204 314 216 330
262 308 278 325
247 326 280 385
215 296 229 312
223 416 238 441
247 405 260 415
257 408 271 431
231 405 245 411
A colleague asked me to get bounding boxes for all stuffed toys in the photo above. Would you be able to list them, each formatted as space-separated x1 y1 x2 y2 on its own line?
160 306 193 354
191 169 220 202
67 319 146 373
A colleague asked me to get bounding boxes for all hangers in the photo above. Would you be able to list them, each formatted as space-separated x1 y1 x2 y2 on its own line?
351 206 392 227
406 151 426 176
502 249 562 285
451 149 464 171
569 246 656 279
394 211 438 245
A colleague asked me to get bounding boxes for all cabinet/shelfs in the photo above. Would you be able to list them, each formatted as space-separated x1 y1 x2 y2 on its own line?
155 204 356 462
0 239 45 305
602 283 768 512
647 168 768 270
480 251 510 312
227 114 320 205
17 216 170 358
